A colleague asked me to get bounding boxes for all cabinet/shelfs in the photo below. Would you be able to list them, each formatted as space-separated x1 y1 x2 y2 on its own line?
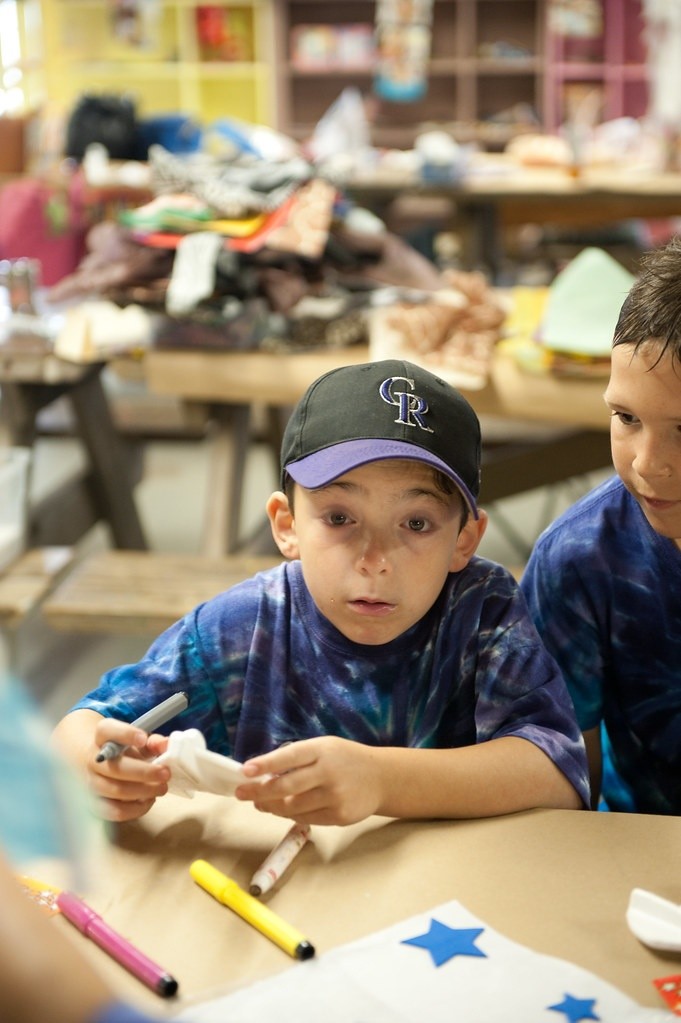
20 2 280 180
280 4 678 165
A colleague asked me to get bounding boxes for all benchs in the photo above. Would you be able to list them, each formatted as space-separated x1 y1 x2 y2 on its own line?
2 537 526 677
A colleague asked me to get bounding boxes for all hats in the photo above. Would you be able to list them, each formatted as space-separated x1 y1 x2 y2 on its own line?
278 359 481 521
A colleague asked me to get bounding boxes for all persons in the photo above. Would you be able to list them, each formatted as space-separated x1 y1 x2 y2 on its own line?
514 232 681 818
48 360 593 829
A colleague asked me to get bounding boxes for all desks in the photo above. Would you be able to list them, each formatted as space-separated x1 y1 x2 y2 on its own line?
96 280 641 561
16 782 681 1023
307 134 681 274
0 298 159 562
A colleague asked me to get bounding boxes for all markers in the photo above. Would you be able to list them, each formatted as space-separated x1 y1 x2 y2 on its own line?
96 691 191 762
57 892 178 997
249 824 312 897
189 858 314 960
